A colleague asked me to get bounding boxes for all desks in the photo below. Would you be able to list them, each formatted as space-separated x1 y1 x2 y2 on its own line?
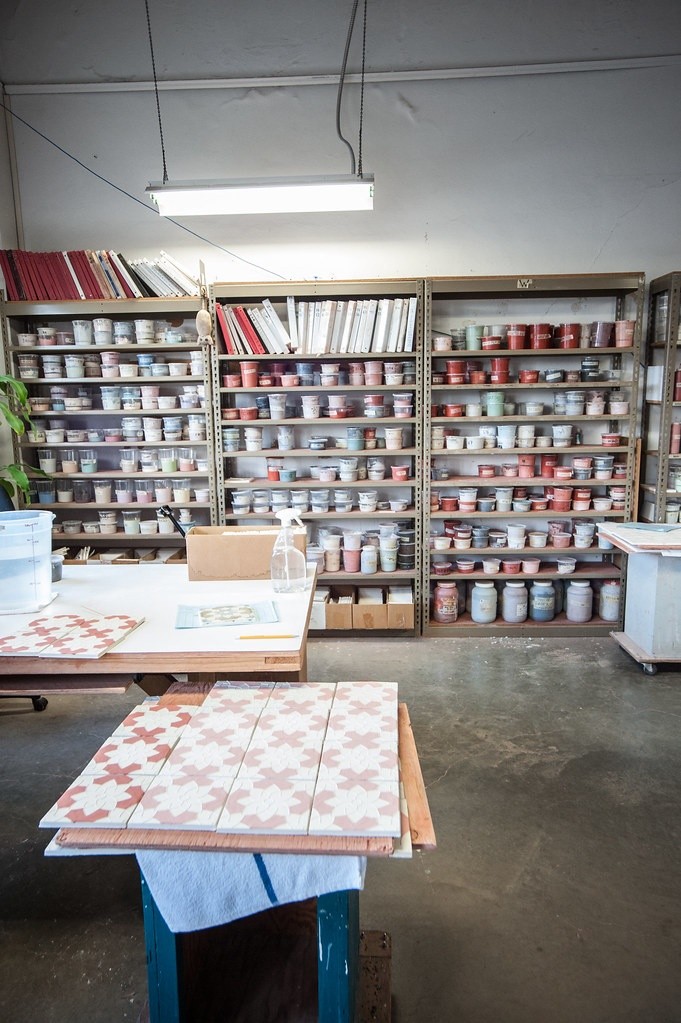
594 521 681 676
38 680 436 1023
0 561 320 697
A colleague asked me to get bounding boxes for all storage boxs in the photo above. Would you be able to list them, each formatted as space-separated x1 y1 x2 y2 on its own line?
86 547 119 564
325 587 354 630
62 550 90 565
166 550 187 564
308 596 326 630
387 598 414 630
352 593 388 630
185 525 307 582
139 548 176 564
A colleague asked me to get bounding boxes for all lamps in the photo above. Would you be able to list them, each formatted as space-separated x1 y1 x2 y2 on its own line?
144 0 375 217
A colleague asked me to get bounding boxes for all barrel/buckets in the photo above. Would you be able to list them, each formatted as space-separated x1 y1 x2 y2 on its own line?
1 511 54 611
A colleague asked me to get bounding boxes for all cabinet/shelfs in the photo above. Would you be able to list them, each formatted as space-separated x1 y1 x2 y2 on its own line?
208 276 424 639
422 271 646 638
638 271 681 524
0 259 218 540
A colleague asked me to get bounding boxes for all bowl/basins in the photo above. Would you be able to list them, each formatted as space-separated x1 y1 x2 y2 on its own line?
18 318 627 575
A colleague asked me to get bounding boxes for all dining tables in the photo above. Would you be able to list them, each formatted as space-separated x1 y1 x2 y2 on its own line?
111 549 153 564
